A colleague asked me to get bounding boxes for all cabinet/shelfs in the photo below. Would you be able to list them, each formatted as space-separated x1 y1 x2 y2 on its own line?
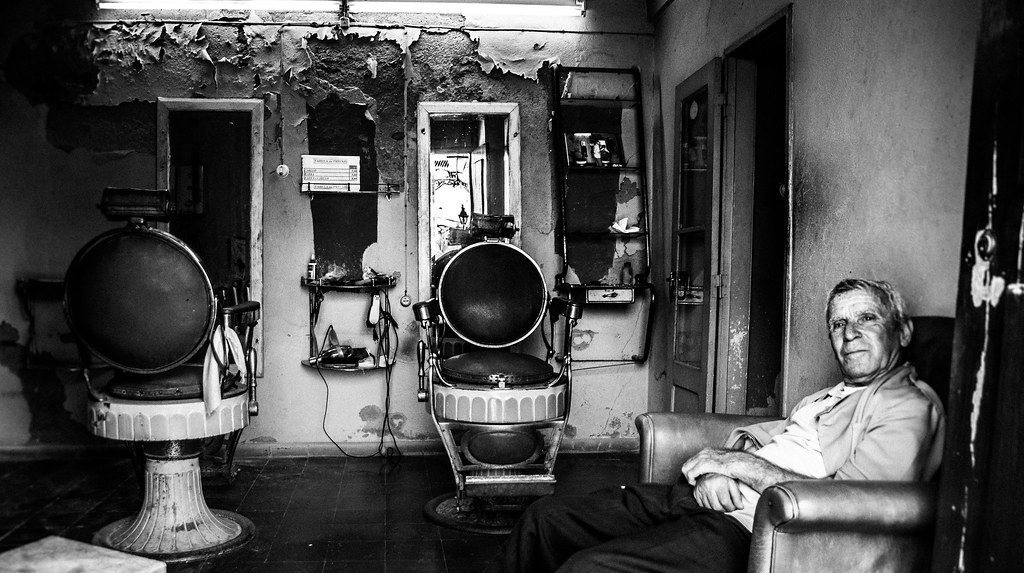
18 275 111 379
549 63 651 303
302 275 397 371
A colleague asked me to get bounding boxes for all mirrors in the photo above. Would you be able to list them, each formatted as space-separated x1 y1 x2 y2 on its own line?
417 102 522 377
155 98 265 378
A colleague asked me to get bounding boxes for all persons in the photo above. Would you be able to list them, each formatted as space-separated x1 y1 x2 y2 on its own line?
486 277 947 573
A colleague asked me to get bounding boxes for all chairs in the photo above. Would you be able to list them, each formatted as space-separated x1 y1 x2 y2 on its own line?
61 188 260 567
411 213 583 535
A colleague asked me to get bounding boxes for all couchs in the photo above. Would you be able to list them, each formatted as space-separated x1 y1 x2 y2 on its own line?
634 316 956 573
